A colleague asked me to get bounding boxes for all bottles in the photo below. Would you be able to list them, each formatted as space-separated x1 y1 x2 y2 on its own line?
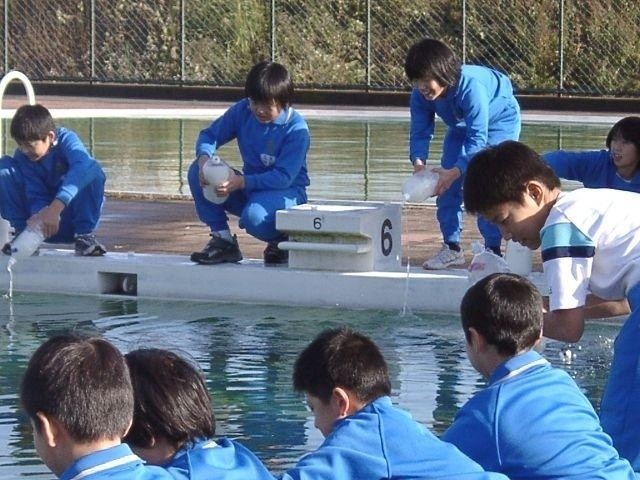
202 153 230 205
468 239 512 286
7 206 61 265
402 165 440 204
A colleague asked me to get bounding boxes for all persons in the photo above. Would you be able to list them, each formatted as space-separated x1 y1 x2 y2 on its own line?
463 140 639 472
537 116 640 193
187 61 310 267
440 270 639 480
121 345 279 480
19 323 188 480
0 104 107 257
274 323 514 480
405 37 523 272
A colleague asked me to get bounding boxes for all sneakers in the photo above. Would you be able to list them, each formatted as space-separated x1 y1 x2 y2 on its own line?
422 242 465 269
264 238 288 263
75 234 106 256
191 234 242 263
